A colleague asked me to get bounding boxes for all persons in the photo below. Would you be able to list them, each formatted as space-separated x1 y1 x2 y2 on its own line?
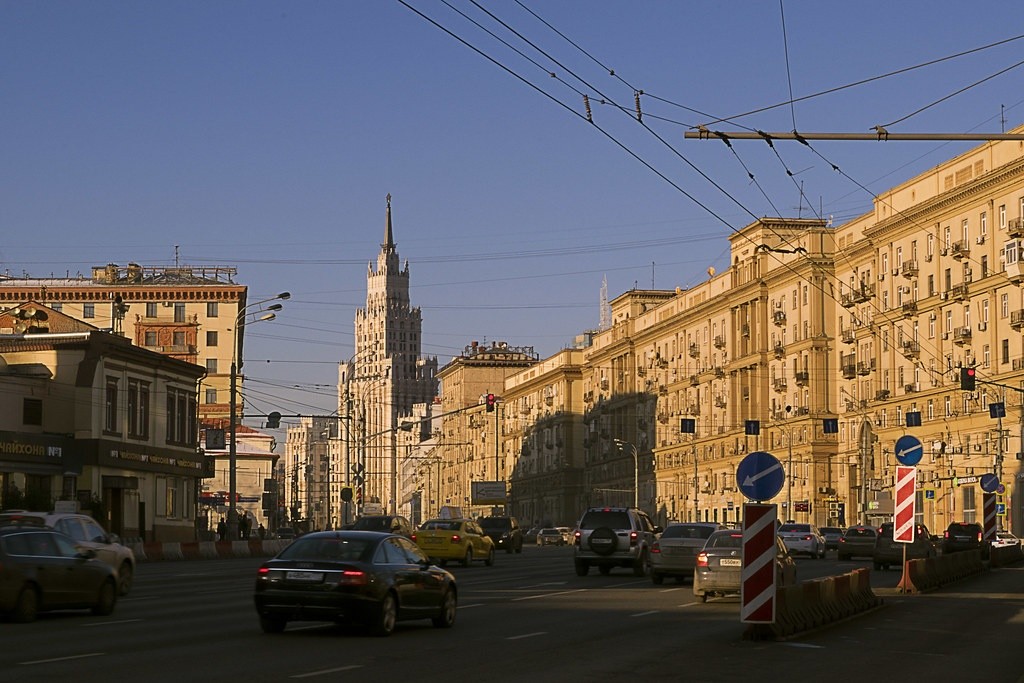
217 514 264 541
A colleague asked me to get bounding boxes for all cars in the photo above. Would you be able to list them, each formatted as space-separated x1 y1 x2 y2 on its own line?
872 519 935 568
692 526 797 602
0 508 136 596
820 527 853 549
0 525 121 615
352 515 417 542
944 521 987 551
648 522 731 584
525 526 576 546
837 525 880 560
978 531 1022 548
777 522 826 558
414 505 496 567
252 529 460 637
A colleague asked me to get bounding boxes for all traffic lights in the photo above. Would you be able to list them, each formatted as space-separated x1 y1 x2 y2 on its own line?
960 367 976 392
356 485 363 506
485 393 495 413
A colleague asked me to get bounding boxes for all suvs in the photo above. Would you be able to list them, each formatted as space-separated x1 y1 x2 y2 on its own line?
479 514 524 554
573 505 663 578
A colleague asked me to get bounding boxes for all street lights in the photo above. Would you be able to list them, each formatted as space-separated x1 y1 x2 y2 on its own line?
229 290 292 540
614 438 640 509
676 431 698 524
356 364 396 517
770 412 793 524
343 333 382 527
840 385 868 527
